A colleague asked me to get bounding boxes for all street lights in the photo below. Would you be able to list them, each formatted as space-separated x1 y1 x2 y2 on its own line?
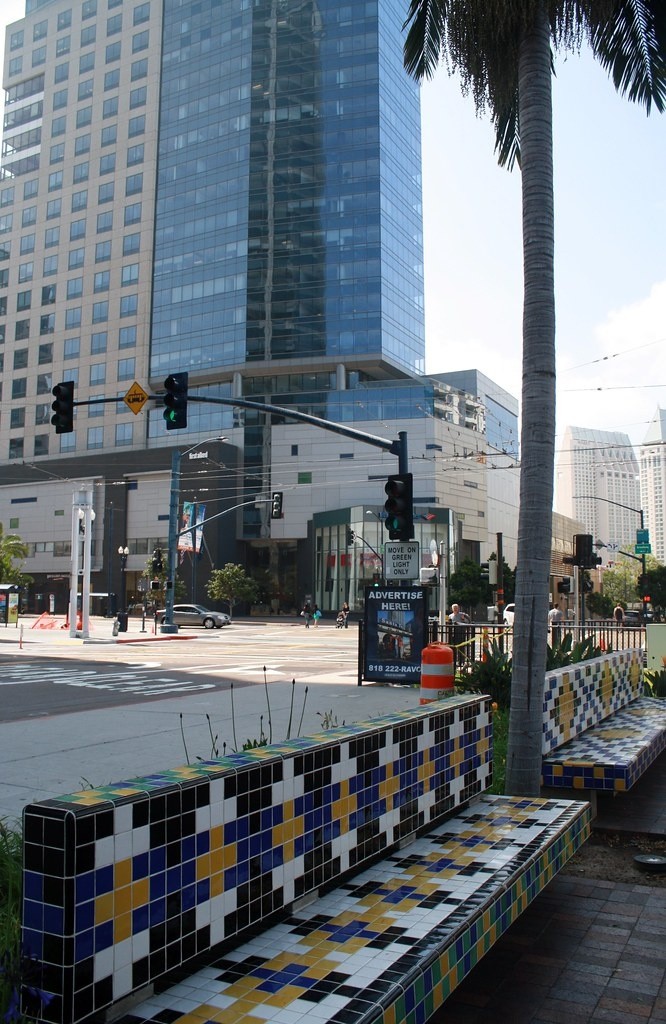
79 508 95 614
117 546 130 632
572 496 647 627
366 510 384 585
161 436 229 633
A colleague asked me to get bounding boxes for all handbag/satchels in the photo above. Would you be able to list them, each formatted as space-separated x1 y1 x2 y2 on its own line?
300 610 305 616
316 609 323 617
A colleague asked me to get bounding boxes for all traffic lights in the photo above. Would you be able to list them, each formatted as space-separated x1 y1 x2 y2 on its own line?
562 577 574 593
347 530 354 545
52 381 74 434
373 572 380 586
385 473 415 540
164 372 188 431
481 561 498 585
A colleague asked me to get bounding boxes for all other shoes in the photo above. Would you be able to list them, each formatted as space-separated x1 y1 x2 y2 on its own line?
314 626 318 629
305 624 309 628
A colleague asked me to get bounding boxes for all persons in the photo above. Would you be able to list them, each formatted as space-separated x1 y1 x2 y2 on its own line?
448 604 470 653
303 603 313 628
313 605 322 627
547 603 563 633
269 606 273 616
614 603 624 633
20 608 25 614
337 602 349 628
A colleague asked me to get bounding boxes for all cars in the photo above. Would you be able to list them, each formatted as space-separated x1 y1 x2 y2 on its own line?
622 610 641 627
156 604 231 629
644 611 657 623
503 603 515 631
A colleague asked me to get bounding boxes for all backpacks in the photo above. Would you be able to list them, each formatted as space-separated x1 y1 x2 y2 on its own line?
615 608 624 620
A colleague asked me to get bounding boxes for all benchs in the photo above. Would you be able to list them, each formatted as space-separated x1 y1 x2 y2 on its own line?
542 649 666 791
20 693 593 1024
